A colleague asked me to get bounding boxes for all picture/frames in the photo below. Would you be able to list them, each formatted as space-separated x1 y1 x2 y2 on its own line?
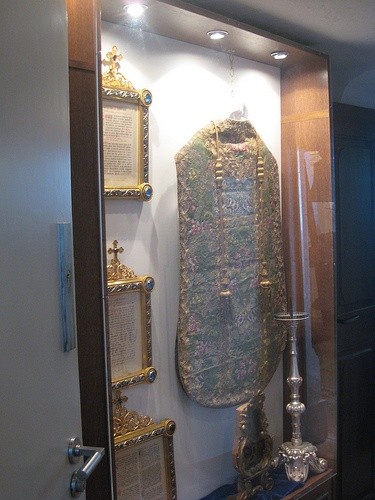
107 239 157 390
101 46 154 199
111 390 179 500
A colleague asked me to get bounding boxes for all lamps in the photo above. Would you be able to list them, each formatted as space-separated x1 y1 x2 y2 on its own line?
272 52 288 60
207 29 227 40
125 4 146 17
